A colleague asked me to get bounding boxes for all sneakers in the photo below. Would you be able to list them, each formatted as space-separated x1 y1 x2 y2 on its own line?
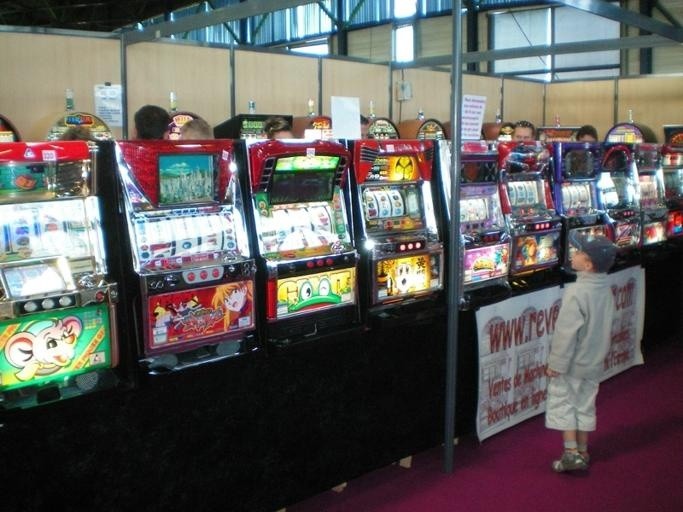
552 451 590 472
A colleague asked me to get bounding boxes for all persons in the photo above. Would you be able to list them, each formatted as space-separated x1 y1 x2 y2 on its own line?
513 120 536 145
359 115 369 140
543 228 617 473
262 117 293 140
178 119 213 141
132 105 172 143
575 125 598 142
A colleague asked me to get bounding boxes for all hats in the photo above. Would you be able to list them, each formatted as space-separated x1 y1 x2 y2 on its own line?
568 229 616 272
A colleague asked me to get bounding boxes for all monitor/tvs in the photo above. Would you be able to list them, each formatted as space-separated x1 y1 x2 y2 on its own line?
362 184 424 234
0 257 75 295
639 173 660 208
508 181 540 209
155 152 220 207
663 168 683 200
265 154 336 207
561 183 592 216
459 188 498 234
601 178 634 208
